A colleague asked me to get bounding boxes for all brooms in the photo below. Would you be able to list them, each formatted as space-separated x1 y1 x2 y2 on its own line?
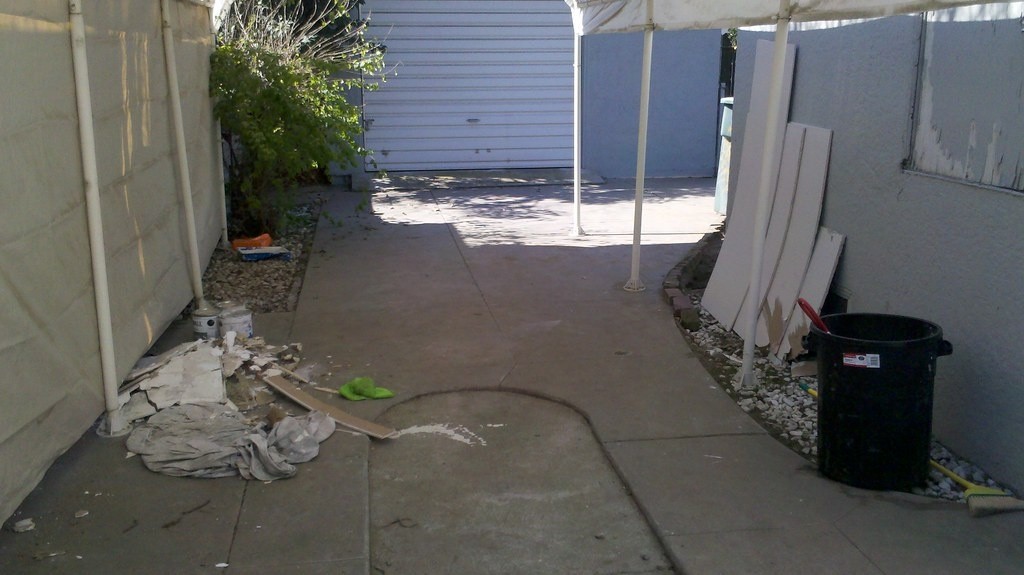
798 381 1024 517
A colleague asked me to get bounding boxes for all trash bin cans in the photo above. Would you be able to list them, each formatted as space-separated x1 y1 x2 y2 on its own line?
800 311 953 493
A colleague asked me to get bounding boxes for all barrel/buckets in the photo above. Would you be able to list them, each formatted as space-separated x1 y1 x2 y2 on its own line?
803 313 954 491
192 300 253 341
